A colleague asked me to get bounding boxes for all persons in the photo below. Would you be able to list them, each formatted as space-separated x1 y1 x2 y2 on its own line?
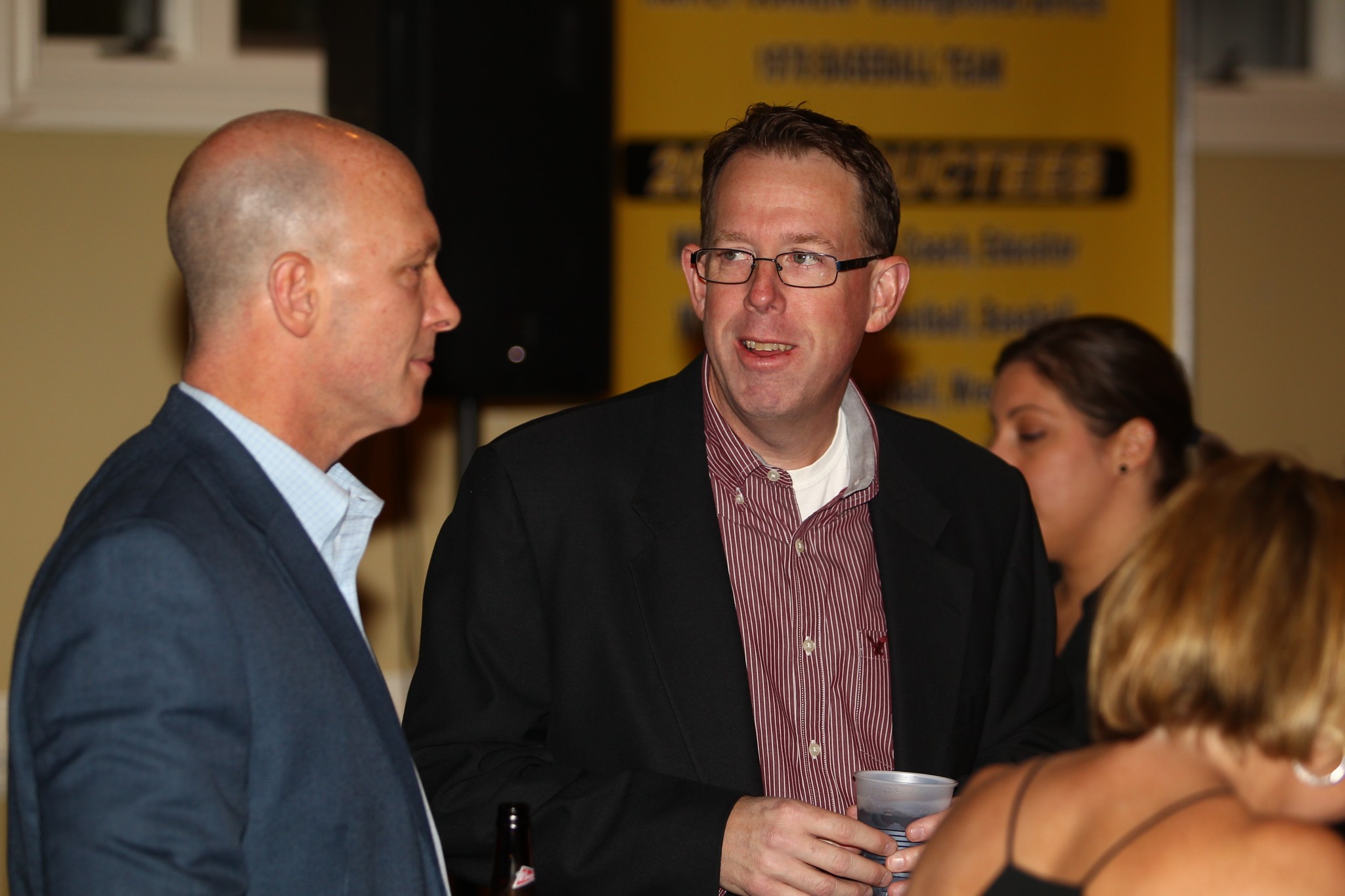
6 107 484 896
903 448 1345 895
402 101 1066 896
988 314 1232 753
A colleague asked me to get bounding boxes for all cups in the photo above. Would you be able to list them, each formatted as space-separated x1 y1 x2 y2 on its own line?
853 768 959 896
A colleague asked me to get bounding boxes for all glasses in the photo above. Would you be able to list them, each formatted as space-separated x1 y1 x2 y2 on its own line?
690 247 894 289
